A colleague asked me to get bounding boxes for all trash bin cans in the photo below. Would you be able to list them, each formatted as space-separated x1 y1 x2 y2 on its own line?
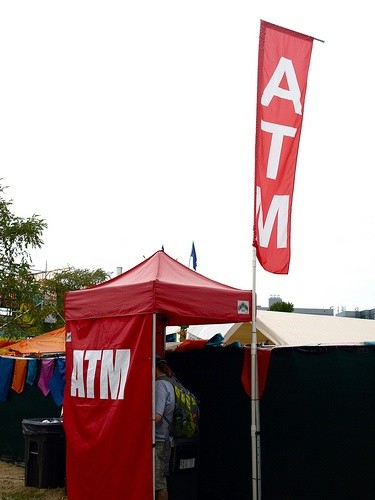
21 418 67 490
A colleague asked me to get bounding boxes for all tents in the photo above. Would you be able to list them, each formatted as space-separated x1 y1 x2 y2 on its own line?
0 247 374 499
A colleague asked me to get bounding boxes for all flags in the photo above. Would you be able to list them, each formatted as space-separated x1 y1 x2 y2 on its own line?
189 241 198 270
257 20 318 279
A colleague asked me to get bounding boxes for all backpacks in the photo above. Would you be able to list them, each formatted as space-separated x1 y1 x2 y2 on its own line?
155 375 200 444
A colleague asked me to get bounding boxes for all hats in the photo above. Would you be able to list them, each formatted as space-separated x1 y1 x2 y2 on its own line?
154 355 167 368
